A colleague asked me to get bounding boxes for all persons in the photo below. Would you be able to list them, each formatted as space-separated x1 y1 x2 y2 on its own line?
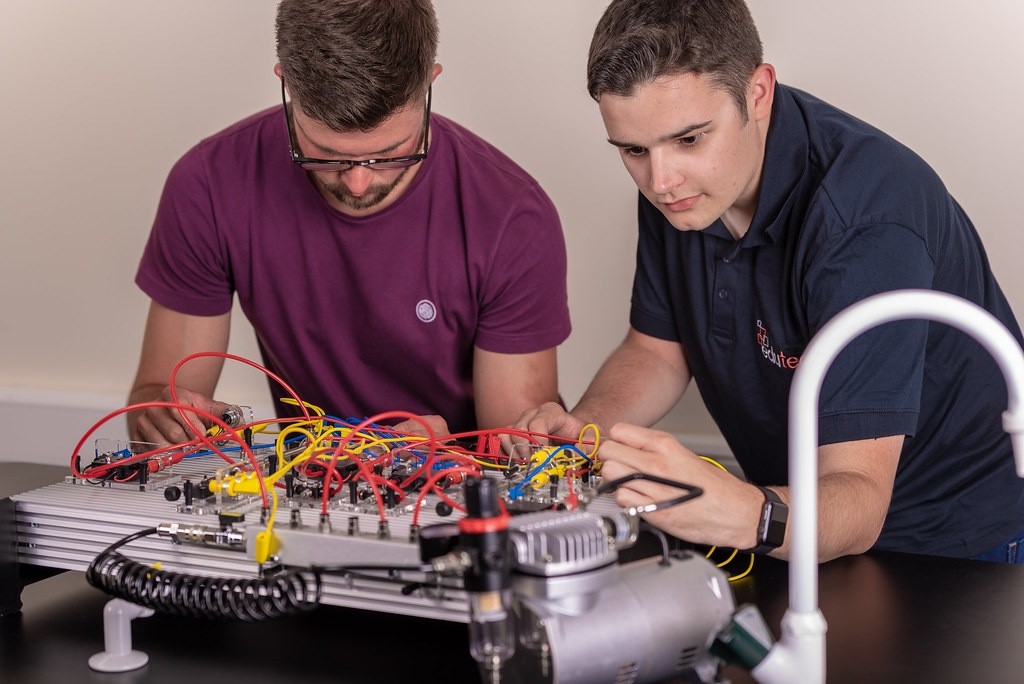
125 0 573 465
492 0 1024 564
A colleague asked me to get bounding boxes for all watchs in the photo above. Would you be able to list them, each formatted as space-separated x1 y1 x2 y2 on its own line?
736 485 789 559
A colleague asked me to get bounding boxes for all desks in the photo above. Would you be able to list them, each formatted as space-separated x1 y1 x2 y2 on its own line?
1 452 1023 684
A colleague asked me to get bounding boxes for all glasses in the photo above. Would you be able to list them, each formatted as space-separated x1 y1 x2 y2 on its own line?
281 73 431 173
